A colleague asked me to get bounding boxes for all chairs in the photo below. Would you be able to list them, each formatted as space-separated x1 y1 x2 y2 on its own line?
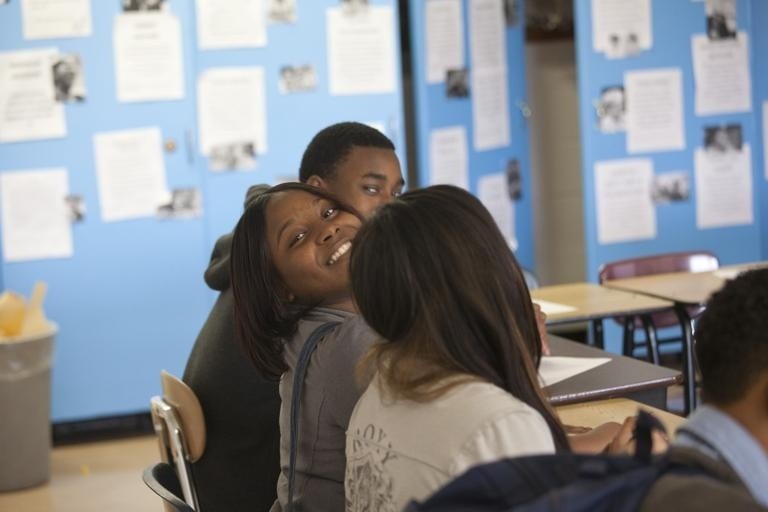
599 250 720 364
144 369 208 511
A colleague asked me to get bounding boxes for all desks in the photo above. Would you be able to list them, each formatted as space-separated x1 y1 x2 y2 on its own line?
551 396 688 456
528 282 675 363
604 261 768 414
534 330 682 410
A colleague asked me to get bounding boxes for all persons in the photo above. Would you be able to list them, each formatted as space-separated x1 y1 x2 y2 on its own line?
339 183 667 511
151 120 406 511
223 178 387 512
404 266 768 510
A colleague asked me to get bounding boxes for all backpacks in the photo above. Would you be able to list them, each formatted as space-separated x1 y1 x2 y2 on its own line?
401 454 695 512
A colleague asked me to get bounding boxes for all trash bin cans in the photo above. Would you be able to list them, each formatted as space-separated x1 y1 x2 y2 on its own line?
0 327 57 495
